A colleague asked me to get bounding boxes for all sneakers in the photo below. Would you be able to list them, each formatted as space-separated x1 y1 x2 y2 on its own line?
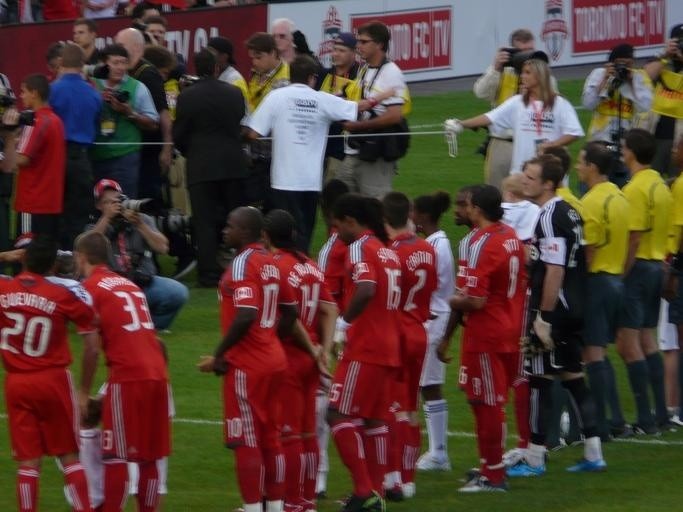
506 458 546 478
501 447 527 466
565 457 608 474
553 409 682 453
382 451 507 502
341 491 385 512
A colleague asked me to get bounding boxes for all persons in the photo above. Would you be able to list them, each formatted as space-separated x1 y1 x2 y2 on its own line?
580 43 654 144
0 1 412 332
643 23 683 148
471 27 560 185
451 58 587 187
196 129 683 512
74 231 173 512
0 232 102 511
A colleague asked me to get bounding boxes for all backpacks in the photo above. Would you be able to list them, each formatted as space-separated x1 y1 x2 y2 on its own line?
371 118 410 162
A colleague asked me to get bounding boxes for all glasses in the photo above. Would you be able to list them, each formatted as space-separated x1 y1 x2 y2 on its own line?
357 39 374 44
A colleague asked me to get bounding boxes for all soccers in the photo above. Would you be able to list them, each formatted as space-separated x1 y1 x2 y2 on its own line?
559 411 570 435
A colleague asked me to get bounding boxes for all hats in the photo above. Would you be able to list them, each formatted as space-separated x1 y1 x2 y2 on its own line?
207 37 236 64
93 178 121 199
263 209 298 248
334 33 356 48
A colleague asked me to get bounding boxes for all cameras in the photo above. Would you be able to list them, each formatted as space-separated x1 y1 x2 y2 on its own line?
117 194 158 218
611 62 630 81
103 88 131 103
0 95 36 130
79 62 110 80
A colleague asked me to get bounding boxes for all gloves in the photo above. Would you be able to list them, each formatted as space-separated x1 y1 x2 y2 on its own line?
528 308 556 356
